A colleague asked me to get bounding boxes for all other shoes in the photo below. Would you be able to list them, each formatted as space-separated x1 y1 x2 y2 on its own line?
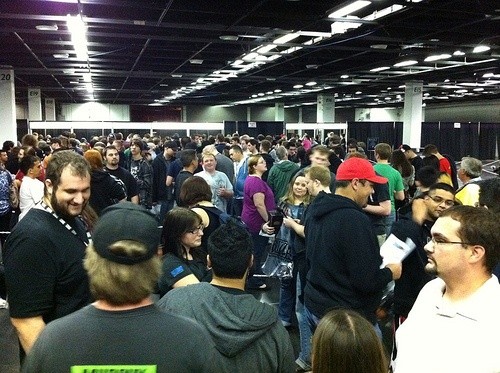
259 285 271 290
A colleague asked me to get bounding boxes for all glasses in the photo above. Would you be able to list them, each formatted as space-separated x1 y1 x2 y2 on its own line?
186 223 205 234
428 194 454 206
428 235 483 245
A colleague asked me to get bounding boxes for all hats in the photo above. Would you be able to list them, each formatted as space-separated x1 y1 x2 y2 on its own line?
164 141 181 149
336 157 387 184
397 145 411 153
92 202 162 264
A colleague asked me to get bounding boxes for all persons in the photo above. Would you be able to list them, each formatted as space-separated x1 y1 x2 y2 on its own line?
159 218 296 373
3 149 97 367
390 205 500 373
307 309 389 372
0 131 499 315
23 201 228 372
305 156 402 348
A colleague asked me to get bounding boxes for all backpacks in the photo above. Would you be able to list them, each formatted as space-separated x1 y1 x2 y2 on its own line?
199 205 251 233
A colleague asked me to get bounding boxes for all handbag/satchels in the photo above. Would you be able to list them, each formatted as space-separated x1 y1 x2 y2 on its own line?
261 237 295 279
